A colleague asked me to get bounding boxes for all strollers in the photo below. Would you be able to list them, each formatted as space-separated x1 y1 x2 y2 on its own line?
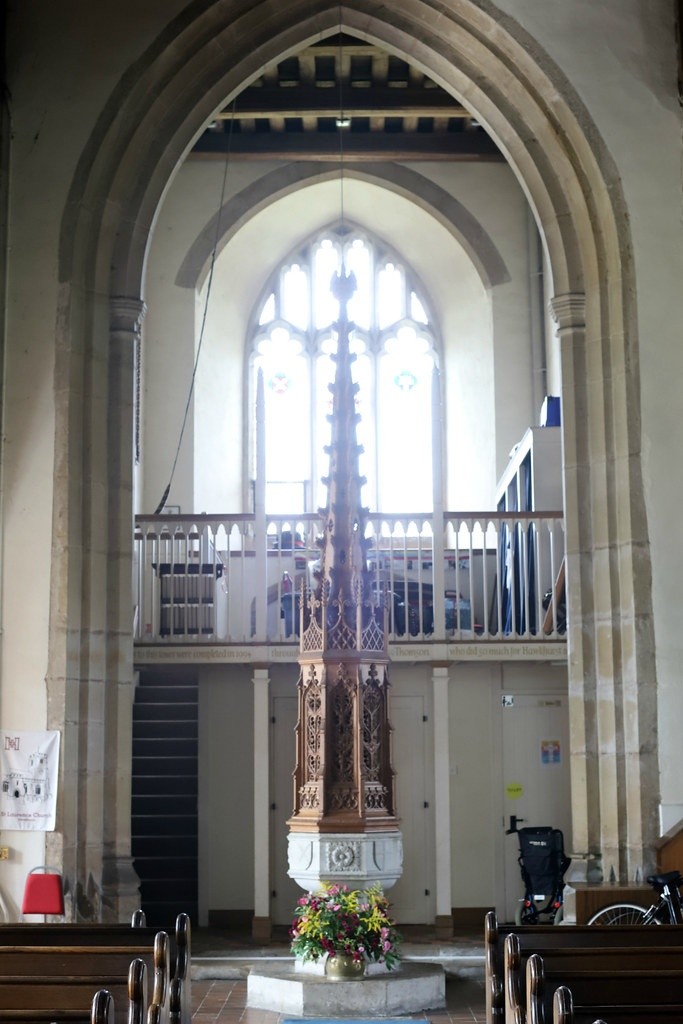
505 826 570 924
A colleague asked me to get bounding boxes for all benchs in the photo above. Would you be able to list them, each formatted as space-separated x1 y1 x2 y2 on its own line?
0 910 192 1024
484 911 683 1024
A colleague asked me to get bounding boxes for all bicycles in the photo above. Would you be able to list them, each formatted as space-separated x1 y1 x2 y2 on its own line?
586 869 683 926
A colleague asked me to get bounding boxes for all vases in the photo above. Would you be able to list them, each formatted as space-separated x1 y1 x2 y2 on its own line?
323 950 366 981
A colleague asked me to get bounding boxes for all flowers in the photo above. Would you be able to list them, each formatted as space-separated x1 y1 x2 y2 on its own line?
288 882 401 970
